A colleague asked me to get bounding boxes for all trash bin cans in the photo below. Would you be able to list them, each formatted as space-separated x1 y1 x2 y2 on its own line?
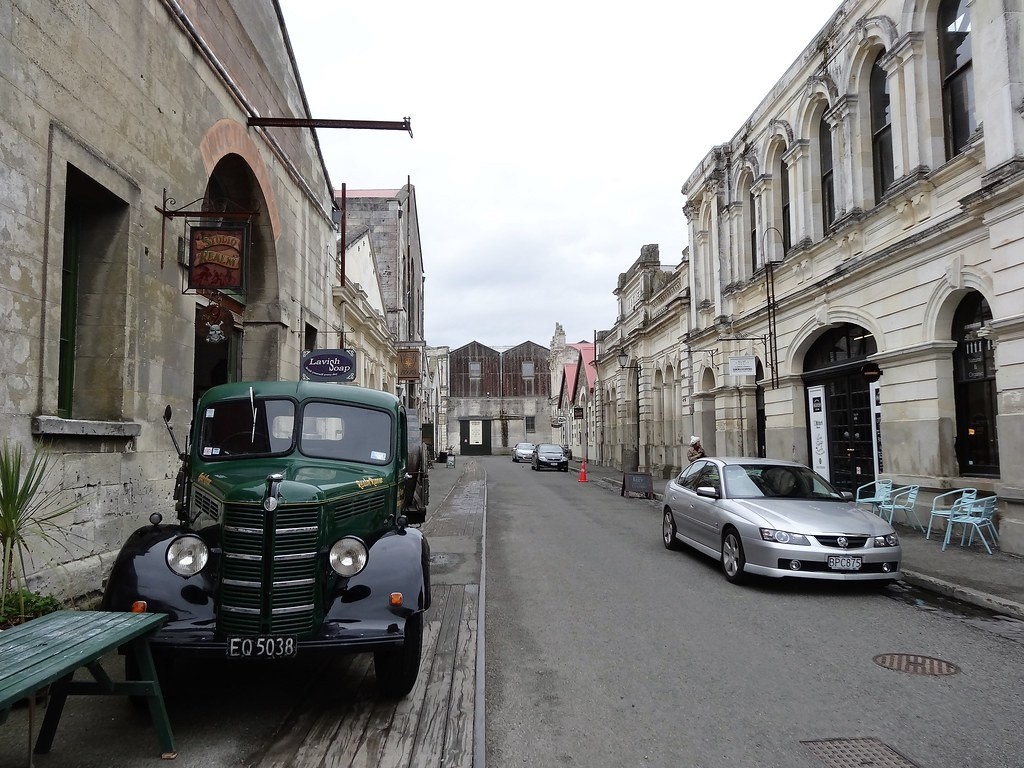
439 451 448 463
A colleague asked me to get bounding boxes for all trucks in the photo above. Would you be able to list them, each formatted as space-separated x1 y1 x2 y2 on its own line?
100 381 431 700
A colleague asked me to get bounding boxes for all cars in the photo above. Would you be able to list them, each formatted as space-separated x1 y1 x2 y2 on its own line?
531 443 569 472
511 443 536 463
662 457 901 588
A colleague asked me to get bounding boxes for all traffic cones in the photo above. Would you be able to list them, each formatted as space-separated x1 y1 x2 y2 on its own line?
579 458 588 482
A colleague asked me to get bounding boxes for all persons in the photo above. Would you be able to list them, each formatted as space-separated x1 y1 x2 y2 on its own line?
687 436 709 463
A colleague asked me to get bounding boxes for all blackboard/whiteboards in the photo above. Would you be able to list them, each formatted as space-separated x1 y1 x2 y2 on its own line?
624 472 653 493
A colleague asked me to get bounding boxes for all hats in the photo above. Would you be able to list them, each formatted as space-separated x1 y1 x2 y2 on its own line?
689 436 700 447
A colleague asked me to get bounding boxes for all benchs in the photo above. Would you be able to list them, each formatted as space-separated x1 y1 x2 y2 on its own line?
0 609 176 752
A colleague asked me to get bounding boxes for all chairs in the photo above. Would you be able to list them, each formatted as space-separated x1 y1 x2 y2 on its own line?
878 484 926 533
941 495 999 554
926 487 987 545
855 478 892 522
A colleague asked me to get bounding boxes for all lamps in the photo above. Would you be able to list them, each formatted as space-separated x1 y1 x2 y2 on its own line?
615 346 641 371
